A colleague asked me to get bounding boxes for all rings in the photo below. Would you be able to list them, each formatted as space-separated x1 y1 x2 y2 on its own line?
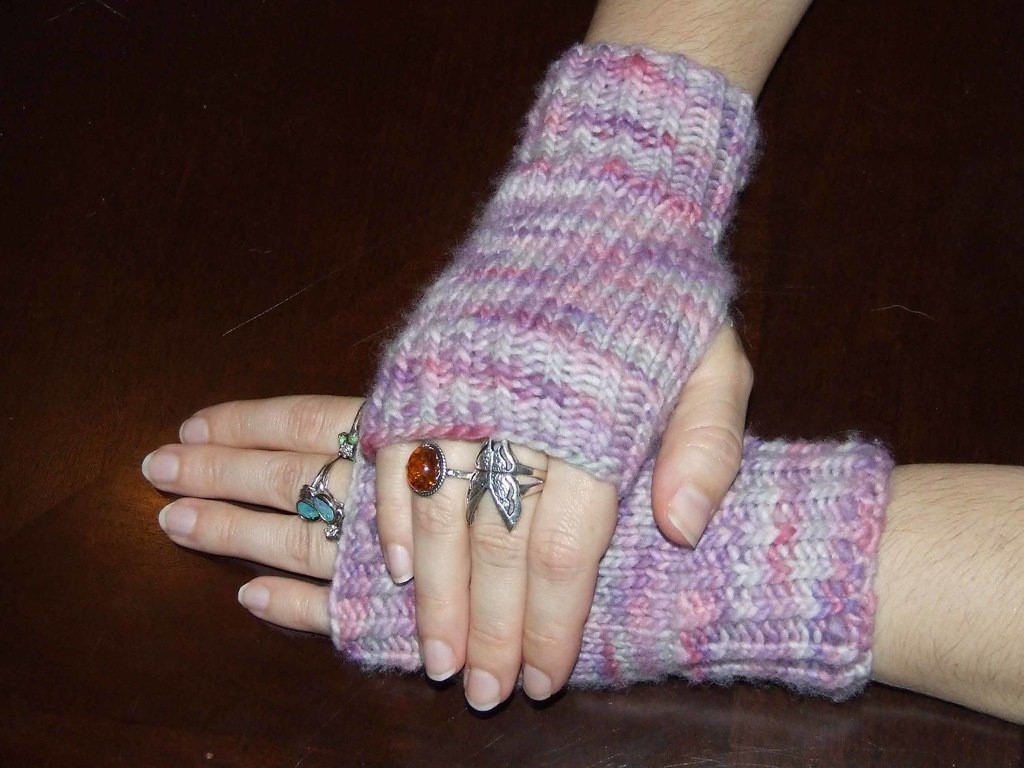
403 442 461 494
295 456 355 541
335 398 363 459
464 436 549 529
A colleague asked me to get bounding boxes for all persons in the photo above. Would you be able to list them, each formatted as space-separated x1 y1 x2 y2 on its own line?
138 1 1021 727
